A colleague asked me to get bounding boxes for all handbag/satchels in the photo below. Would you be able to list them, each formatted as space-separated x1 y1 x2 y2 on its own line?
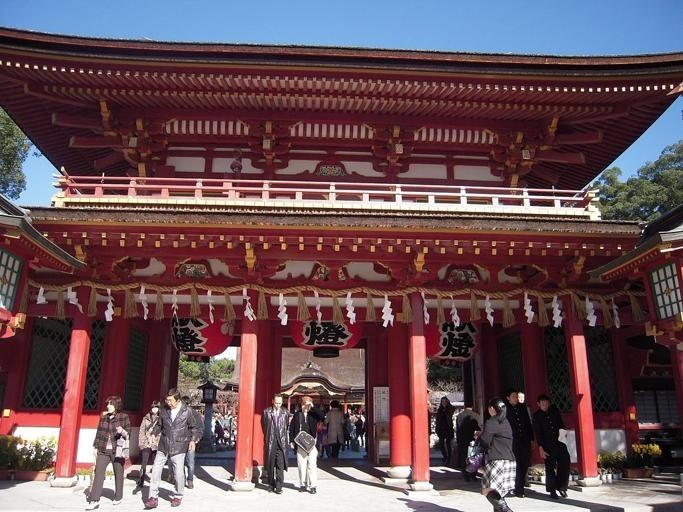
115 432 130 458
294 431 316 453
465 452 484 473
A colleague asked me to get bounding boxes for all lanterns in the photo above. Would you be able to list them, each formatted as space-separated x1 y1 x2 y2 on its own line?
170 317 234 363
291 320 363 358
424 320 482 367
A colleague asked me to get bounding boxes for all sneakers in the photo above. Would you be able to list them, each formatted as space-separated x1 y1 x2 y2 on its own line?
86 479 193 509
516 479 568 498
267 483 317 494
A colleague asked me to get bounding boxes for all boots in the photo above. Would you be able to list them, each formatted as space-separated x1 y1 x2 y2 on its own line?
137 469 146 486
486 491 514 512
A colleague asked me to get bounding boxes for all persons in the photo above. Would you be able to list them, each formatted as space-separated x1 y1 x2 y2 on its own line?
88 392 231 510
427 392 571 511
261 393 366 495
